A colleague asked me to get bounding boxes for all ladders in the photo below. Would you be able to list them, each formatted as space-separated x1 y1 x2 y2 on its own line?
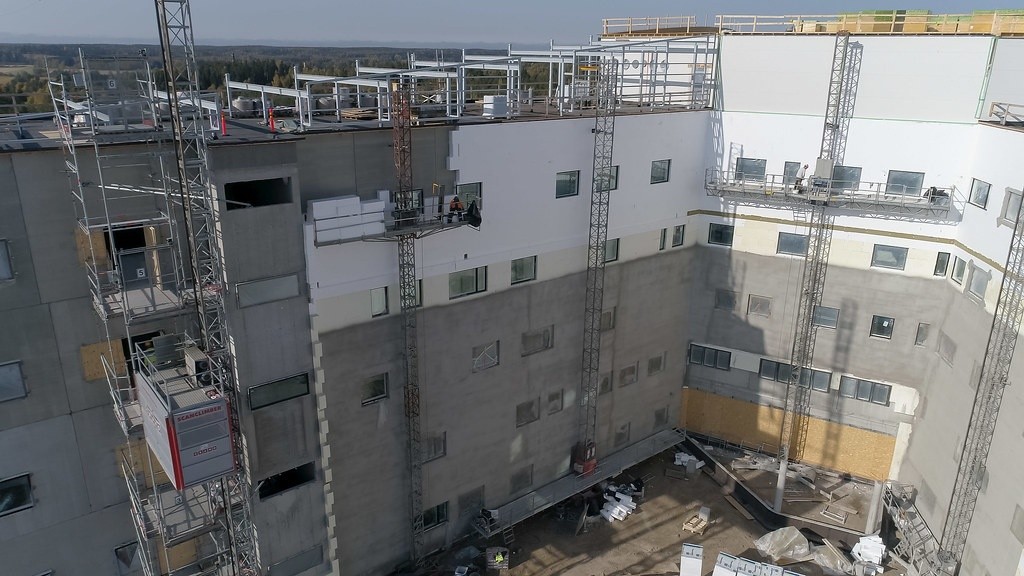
503 526 516 544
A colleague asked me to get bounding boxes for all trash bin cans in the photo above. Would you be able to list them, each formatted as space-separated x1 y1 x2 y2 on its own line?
551 469 643 520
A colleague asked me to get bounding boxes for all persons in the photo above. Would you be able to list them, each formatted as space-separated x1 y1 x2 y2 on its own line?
447 196 464 223
794 164 808 190
812 176 829 196
141 338 156 367
